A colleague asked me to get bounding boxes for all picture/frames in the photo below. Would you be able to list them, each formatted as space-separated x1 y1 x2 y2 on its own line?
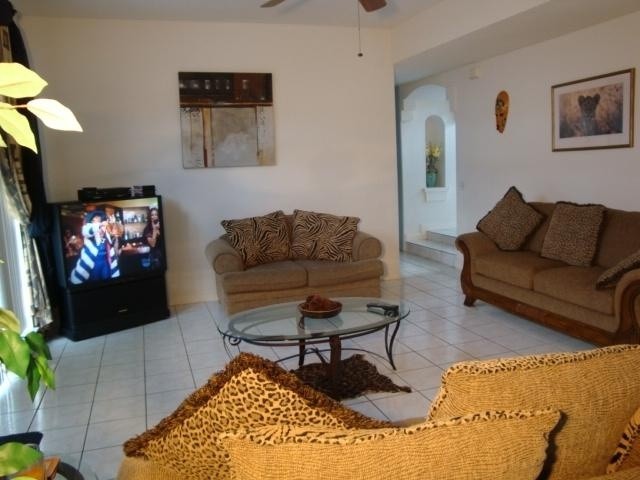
550 67 636 152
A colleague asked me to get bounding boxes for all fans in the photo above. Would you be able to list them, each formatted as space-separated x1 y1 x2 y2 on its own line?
253 0 391 12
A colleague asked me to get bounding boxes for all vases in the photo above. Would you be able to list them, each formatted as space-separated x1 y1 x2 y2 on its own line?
427 171 438 187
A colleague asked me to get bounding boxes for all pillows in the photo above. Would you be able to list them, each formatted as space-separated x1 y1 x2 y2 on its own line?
289 207 360 265
218 210 290 264
540 199 605 267
596 251 639 289
126 354 397 479
425 344 639 479
207 406 561 479
476 186 537 248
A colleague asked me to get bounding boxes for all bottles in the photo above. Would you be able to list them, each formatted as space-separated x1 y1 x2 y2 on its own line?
123 213 146 241
179 79 250 101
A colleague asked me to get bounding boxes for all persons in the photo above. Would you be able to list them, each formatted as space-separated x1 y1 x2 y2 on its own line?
143 208 164 276
69 210 122 285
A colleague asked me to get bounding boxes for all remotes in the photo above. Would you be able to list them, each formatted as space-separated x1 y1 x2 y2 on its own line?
367 301 399 311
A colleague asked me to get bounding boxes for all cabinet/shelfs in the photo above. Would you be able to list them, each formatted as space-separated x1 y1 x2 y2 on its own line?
54 266 171 341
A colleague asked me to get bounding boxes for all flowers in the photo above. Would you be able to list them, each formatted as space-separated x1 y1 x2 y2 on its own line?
426 140 442 171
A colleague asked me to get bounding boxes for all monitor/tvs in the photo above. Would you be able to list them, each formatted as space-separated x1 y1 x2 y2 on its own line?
46 195 167 294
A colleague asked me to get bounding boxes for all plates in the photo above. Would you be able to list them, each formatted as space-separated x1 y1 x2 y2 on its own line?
298 300 342 318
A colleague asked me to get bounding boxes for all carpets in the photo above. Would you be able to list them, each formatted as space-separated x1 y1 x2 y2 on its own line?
290 354 412 402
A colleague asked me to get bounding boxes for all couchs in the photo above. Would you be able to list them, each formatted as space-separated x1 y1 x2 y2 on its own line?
205 208 384 313
455 200 639 345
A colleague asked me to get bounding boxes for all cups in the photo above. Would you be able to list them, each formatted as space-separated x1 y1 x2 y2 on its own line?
137 247 151 268
